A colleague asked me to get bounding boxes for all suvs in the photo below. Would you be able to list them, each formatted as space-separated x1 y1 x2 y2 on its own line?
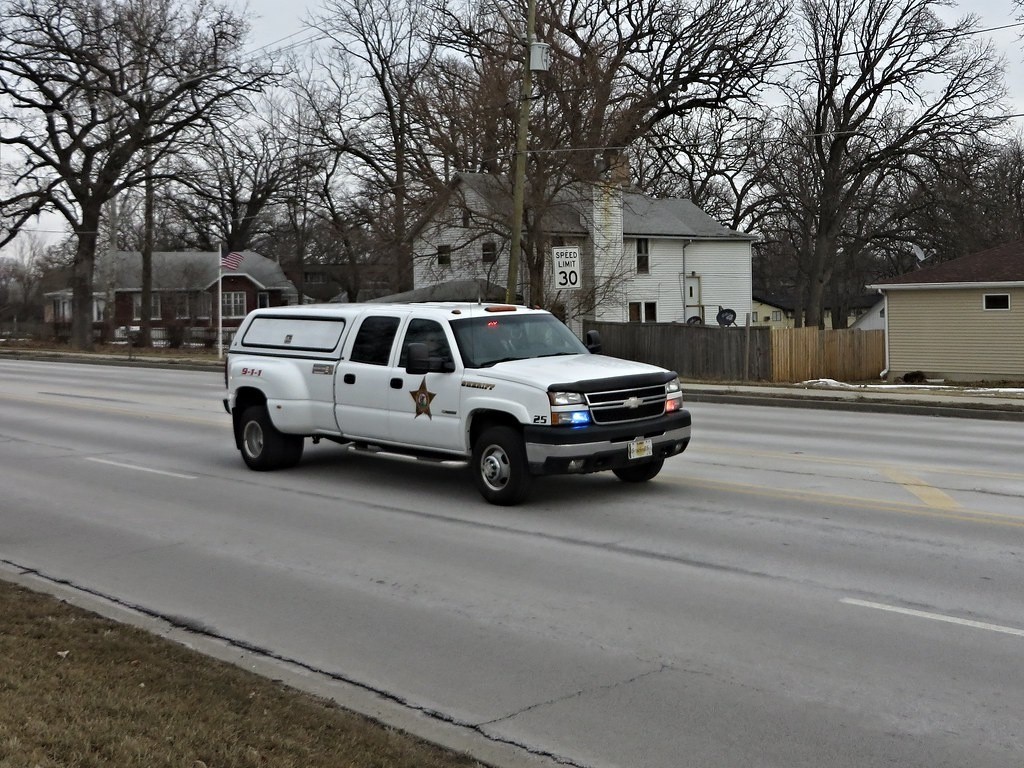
222 298 691 506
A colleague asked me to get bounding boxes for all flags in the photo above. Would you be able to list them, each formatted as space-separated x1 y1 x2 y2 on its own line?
220 246 244 270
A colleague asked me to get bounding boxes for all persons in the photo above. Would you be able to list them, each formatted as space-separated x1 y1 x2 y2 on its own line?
415 329 440 352
494 324 522 352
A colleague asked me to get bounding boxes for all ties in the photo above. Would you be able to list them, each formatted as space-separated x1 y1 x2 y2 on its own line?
507 341 512 350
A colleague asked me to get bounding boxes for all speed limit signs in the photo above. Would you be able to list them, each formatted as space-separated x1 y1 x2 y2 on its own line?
553 245 583 288
